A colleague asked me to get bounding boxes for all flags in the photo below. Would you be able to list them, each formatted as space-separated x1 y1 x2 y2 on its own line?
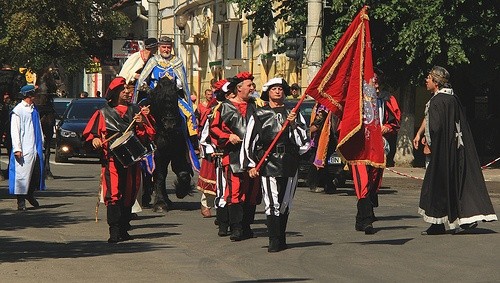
306 7 387 169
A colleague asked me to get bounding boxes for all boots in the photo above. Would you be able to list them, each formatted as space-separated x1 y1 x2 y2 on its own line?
230 204 242 240
281 213 290 249
242 207 257 238
106 206 119 242
357 198 372 233
267 215 279 252
355 199 366 231
120 207 129 241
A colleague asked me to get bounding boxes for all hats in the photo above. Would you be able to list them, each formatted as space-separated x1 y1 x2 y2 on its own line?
20 85 38 92
229 73 254 92
159 36 172 46
105 76 126 101
259 77 292 102
145 37 158 49
214 80 229 94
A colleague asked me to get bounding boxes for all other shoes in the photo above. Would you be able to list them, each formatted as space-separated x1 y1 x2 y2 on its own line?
24 193 39 207
218 224 226 235
17 196 26 210
422 222 445 236
452 221 478 235
201 203 211 217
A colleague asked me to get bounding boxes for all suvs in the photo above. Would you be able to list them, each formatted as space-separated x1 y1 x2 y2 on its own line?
55 98 113 163
52 96 75 139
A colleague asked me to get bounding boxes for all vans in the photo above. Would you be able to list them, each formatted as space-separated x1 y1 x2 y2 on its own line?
284 98 350 188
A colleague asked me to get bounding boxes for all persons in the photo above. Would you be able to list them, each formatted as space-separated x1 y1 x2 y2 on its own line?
350 68 402 235
413 66 498 235
8 85 47 210
0 91 12 155
82 36 333 252
80 92 88 98
